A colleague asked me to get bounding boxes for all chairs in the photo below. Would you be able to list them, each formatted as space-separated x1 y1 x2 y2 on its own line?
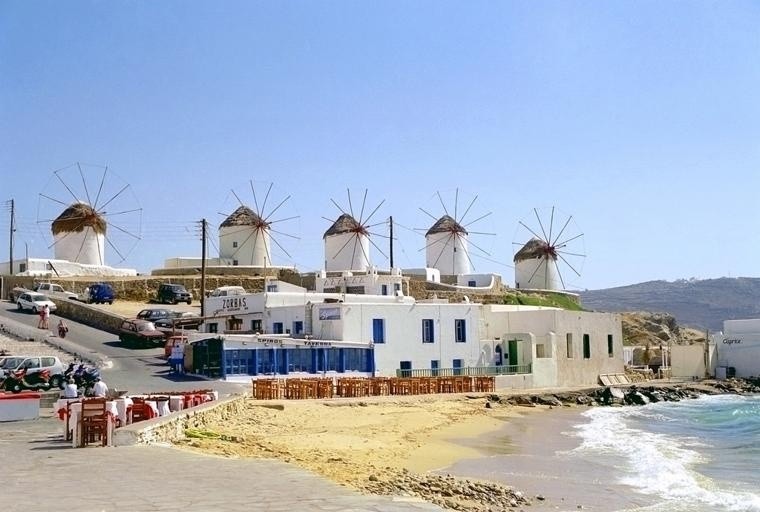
251 377 497 400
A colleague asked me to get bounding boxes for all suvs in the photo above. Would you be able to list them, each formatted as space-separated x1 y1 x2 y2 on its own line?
36 281 78 300
157 283 192 305
136 308 183 329
88 284 114 305
119 318 167 346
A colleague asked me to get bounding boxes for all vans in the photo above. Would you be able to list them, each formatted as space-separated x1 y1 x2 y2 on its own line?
204 286 247 297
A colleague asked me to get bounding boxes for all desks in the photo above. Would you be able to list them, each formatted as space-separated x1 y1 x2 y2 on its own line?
53 384 218 448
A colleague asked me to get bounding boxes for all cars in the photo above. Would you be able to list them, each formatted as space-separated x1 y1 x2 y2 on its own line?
0 357 61 388
16 292 57 314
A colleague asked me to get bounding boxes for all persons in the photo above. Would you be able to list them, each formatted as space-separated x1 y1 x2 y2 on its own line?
43 304 50 329
39 306 45 328
90 377 110 397
58 320 69 338
65 378 78 397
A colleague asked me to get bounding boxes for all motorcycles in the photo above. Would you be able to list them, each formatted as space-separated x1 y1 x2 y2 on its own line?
75 360 101 387
60 357 77 391
8 363 50 394
